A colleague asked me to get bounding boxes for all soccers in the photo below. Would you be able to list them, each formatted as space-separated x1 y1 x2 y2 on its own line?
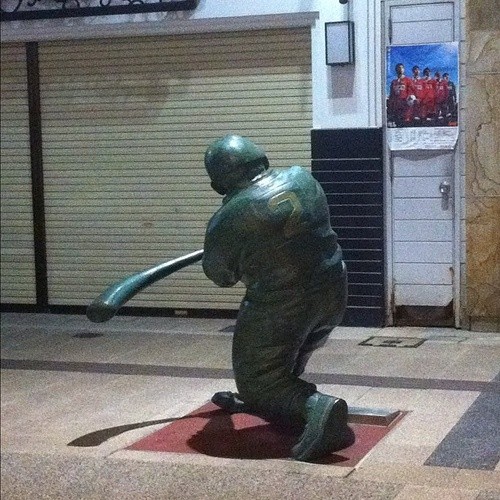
407 95 416 107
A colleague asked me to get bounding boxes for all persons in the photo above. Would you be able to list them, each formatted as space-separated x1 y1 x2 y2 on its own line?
199 134 349 461
389 64 458 127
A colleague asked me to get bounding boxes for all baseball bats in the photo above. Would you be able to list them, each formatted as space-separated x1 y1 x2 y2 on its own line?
87 249 205 324
